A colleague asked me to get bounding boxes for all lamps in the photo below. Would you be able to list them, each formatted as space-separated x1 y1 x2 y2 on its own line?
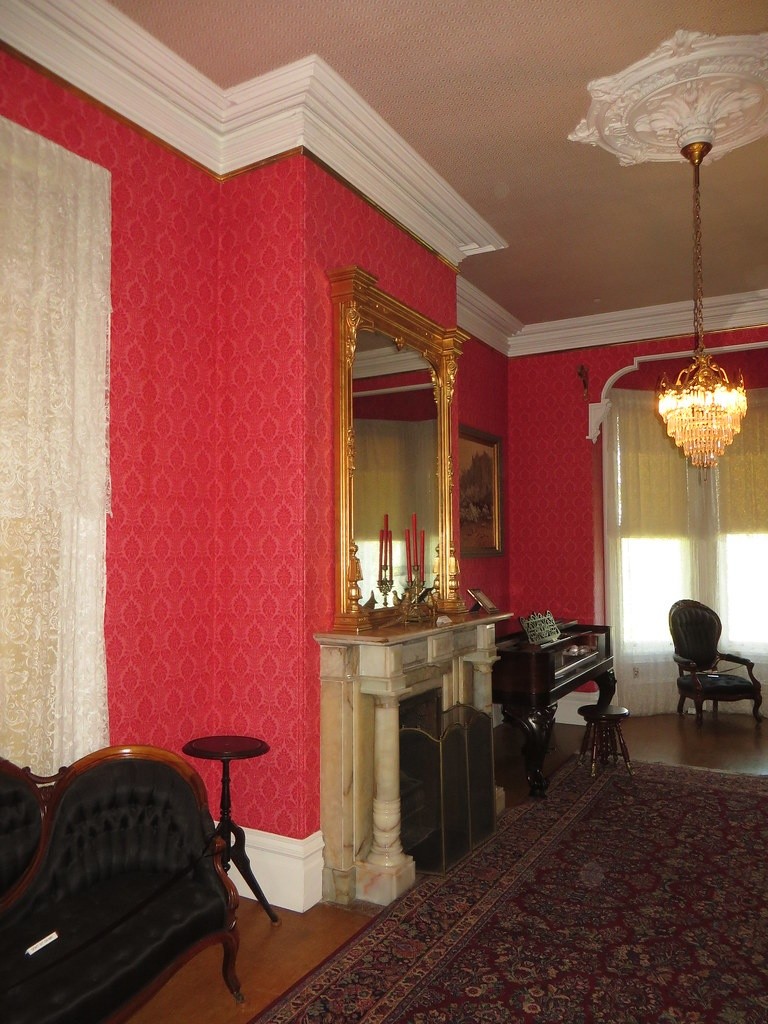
566 30 768 468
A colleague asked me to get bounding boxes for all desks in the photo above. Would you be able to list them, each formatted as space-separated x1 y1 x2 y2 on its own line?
182 736 283 927
493 624 618 801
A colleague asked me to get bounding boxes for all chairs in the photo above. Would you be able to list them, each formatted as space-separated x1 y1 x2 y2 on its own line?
668 600 764 728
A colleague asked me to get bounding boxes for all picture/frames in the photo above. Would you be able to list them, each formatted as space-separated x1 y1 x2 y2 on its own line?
467 589 500 615
457 423 506 557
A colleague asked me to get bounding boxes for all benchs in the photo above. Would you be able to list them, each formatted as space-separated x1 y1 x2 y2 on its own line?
0 745 244 1024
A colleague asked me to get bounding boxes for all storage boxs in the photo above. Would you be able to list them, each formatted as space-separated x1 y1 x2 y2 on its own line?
25 931 60 956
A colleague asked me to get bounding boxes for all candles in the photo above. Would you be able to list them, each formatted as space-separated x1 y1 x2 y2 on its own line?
405 529 412 581
388 531 392 579
384 514 388 565
420 530 425 581
379 530 384 570
411 514 418 566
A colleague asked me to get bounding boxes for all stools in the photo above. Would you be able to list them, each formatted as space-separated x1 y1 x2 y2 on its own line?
578 704 635 777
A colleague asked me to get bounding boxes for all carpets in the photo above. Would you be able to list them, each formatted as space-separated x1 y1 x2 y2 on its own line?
246 754 767 1024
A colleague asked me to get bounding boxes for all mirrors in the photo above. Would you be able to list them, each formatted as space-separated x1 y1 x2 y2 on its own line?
324 264 469 633
